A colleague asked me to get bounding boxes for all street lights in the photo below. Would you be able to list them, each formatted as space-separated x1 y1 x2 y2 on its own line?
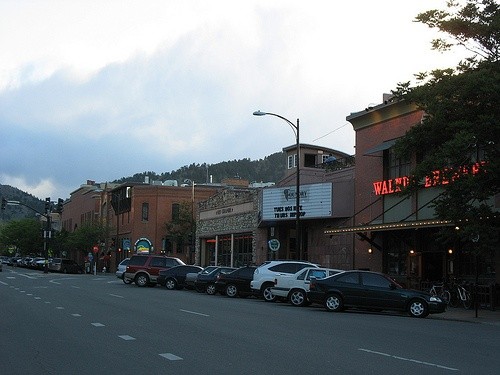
95 189 120 268
253 109 301 263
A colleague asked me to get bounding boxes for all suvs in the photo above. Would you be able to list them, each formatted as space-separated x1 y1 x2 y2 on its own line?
125 254 186 287
250 261 320 301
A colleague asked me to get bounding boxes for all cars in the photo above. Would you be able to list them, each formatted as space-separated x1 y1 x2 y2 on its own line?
271 266 347 306
116 258 132 284
157 265 259 298
0 255 82 274
308 269 446 318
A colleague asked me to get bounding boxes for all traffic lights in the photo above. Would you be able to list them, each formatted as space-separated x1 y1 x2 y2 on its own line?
58 198 64 214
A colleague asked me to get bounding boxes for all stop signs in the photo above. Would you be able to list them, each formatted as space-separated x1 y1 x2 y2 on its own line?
92 246 98 253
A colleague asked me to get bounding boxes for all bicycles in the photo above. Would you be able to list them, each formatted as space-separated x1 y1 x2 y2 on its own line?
428 278 473 310
